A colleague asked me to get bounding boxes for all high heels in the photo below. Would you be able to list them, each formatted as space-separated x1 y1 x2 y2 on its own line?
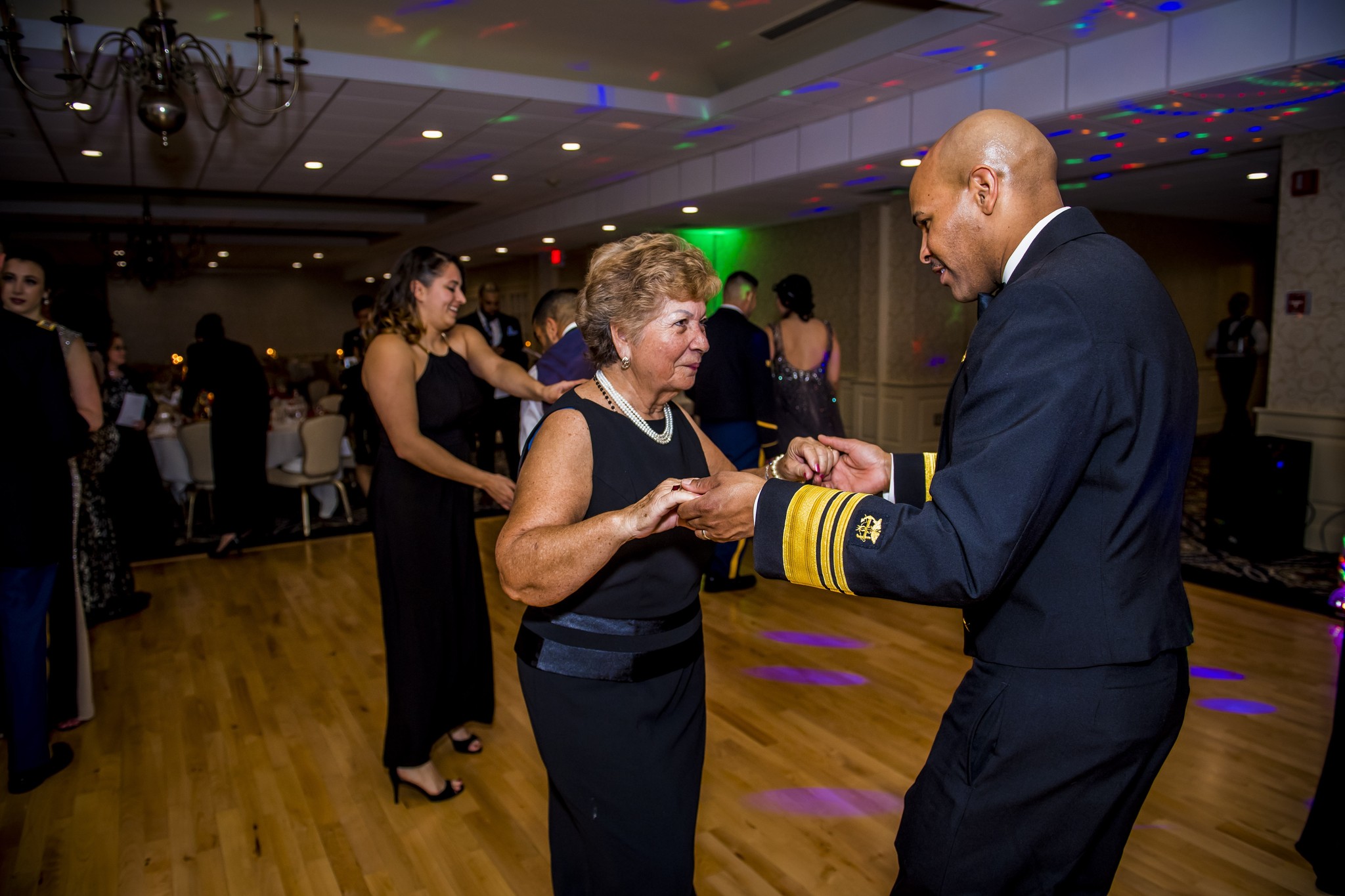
446 732 483 753
207 537 242 558
388 768 464 804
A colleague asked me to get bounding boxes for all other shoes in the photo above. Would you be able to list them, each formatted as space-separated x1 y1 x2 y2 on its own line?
8 742 73 793
704 575 756 593
57 718 79 730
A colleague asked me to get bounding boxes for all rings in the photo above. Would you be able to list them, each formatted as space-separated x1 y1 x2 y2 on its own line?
670 485 682 491
702 529 711 540
828 446 833 451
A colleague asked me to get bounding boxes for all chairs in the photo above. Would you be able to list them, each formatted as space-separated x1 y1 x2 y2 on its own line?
179 421 214 540
271 413 352 536
308 378 330 407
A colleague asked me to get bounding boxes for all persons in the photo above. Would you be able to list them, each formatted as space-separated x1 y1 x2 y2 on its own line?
496 233 843 896
765 276 850 458
684 270 772 593
0 244 589 802
1202 291 1270 437
696 109 1195 896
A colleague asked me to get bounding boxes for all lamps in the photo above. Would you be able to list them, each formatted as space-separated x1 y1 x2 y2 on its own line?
0 0 310 147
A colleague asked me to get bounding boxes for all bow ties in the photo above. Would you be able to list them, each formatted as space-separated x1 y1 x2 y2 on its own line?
977 280 1004 321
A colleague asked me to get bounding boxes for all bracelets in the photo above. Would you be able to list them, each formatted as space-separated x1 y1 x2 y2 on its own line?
764 453 806 485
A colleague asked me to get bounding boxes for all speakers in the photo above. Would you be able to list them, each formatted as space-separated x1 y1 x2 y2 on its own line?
1207 435 1312 559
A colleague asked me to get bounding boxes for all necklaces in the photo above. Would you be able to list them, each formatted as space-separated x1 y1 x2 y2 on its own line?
594 371 675 445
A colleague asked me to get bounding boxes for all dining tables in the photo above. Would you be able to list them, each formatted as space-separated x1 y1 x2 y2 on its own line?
150 418 302 531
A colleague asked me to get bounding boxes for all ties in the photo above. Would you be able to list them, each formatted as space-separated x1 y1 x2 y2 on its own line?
485 321 493 347
